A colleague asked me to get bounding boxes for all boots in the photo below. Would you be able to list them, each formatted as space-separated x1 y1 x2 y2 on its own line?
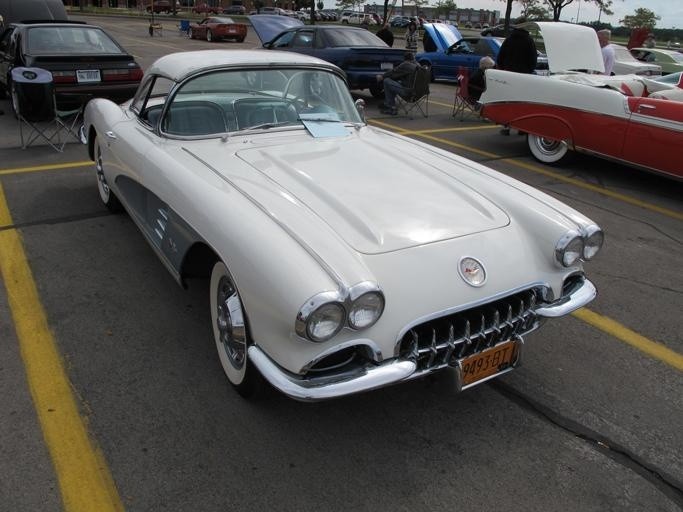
145 1 181 15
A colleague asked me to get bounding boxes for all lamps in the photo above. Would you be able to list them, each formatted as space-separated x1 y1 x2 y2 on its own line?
146 99 227 135
451 64 481 121
176 20 189 34
391 65 433 120
148 18 162 37
233 97 297 130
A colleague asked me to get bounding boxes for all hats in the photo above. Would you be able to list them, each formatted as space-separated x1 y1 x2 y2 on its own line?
609 43 662 77
414 23 549 83
476 22 682 184
187 16 247 43
670 41 681 48
191 4 489 29
480 24 530 38
245 14 413 99
628 48 682 77
79 49 602 405
0 1 144 121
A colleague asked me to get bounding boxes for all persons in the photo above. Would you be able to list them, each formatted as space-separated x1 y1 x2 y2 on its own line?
468 56 495 121
404 21 418 55
376 23 394 47
498 17 537 136
590 29 615 76
423 30 437 52
637 33 656 61
375 52 421 115
